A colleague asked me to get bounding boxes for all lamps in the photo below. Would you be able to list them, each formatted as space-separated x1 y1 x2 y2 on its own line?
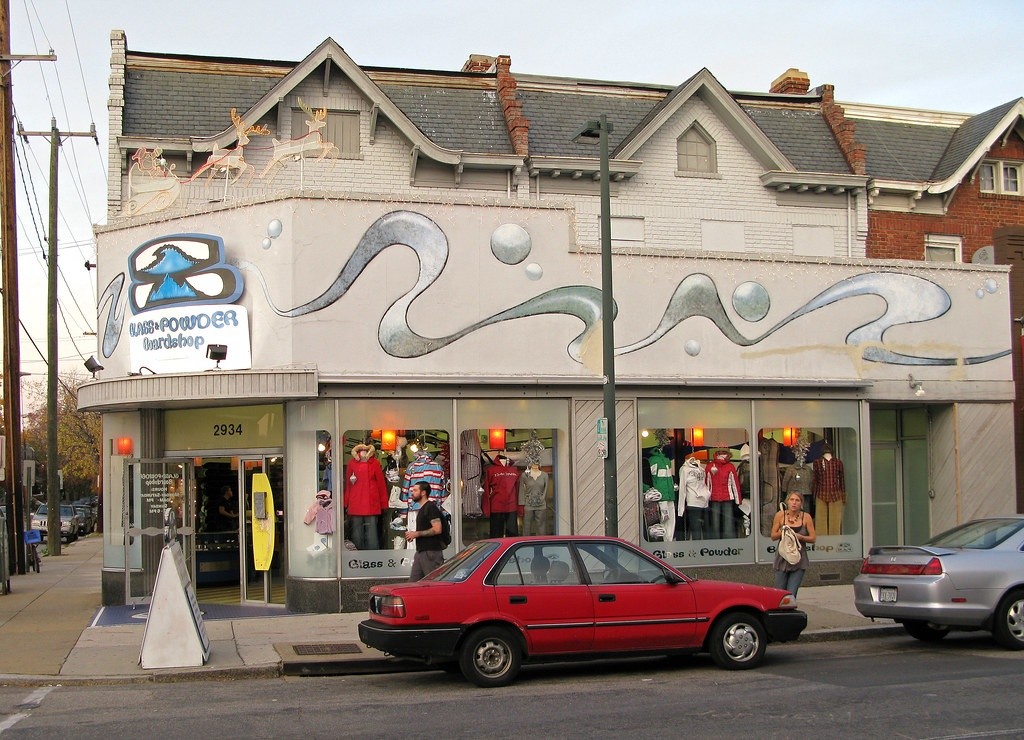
83 355 104 381
205 343 228 369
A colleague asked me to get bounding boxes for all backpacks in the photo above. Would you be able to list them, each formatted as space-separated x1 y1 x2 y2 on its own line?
425 502 452 549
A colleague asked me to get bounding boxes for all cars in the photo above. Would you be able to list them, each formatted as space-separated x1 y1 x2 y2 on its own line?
30 504 79 543
73 505 97 536
357 536 808 690
855 516 1024 652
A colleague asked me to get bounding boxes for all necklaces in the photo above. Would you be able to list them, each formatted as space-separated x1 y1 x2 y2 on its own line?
787 511 799 524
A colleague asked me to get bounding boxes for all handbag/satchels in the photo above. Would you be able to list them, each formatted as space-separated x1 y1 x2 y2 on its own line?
528 495 544 507
778 525 802 565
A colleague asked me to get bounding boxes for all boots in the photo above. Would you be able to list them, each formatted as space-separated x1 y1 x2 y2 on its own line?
393 536 405 549
388 486 409 509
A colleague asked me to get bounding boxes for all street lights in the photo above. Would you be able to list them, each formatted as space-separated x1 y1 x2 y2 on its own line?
567 115 618 568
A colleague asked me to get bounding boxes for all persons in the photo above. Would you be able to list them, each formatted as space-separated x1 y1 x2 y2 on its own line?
678 457 711 540
738 454 750 536
215 486 239 531
782 456 816 520
648 446 676 542
401 451 446 550
405 481 452 582
704 450 742 538
771 491 816 600
343 444 389 550
518 463 549 536
483 455 522 537
814 450 847 536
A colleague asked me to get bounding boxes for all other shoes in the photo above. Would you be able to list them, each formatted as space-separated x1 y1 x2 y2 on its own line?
390 519 407 531
385 466 400 483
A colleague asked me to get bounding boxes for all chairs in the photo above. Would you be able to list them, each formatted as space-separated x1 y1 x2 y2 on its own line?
530 556 550 585
549 561 570 584
